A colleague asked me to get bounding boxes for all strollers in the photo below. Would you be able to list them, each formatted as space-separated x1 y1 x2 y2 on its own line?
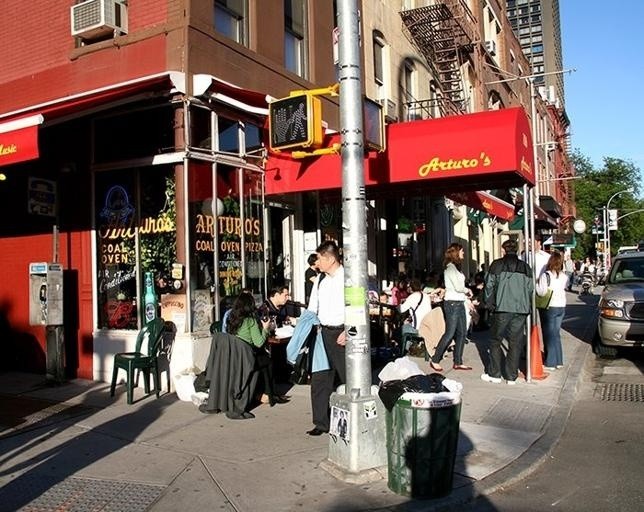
577 271 594 300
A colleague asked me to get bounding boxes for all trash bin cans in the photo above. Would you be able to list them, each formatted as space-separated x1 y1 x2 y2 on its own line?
378 376 463 500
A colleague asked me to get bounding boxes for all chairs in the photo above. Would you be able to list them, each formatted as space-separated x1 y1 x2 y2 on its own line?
191 300 431 420
109 317 166 405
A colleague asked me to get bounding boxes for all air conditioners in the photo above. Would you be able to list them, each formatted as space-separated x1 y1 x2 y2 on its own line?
484 41 496 56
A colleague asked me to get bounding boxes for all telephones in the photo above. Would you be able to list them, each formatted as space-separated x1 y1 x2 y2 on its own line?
40 285 46 302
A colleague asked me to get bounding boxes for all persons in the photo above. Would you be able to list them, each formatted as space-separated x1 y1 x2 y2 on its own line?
481 239 533 381
392 273 446 355
474 264 485 284
304 254 320 309
222 283 298 407
305 240 346 437
521 237 575 372
430 242 474 371
579 256 594 274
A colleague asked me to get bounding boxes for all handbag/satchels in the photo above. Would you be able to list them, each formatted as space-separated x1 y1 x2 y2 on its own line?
293 326 318 386
402 308 416 329
536 287 553 310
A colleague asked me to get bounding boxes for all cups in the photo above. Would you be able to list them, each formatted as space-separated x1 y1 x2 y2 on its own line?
259 315 290 331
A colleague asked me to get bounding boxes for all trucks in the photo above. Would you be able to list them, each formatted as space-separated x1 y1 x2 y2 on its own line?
615 243 641 257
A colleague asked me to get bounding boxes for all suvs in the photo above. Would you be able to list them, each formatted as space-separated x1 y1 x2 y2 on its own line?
592 249 644 362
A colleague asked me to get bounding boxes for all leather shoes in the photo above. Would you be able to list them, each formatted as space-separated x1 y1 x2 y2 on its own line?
430 362 443 372
453 364 472 370
307 428 324 437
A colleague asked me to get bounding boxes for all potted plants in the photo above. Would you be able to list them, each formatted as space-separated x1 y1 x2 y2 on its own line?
69 0 128 41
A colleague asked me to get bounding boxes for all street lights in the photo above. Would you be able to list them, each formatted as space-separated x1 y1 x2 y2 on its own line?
602 186 637 281
265 89 324 155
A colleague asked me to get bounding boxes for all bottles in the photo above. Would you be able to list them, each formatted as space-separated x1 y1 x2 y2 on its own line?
141 271 157 326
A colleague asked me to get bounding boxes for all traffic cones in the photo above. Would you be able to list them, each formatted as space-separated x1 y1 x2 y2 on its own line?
515 325 554 381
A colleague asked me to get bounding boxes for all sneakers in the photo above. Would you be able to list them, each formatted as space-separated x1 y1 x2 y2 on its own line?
481 374 501 383
